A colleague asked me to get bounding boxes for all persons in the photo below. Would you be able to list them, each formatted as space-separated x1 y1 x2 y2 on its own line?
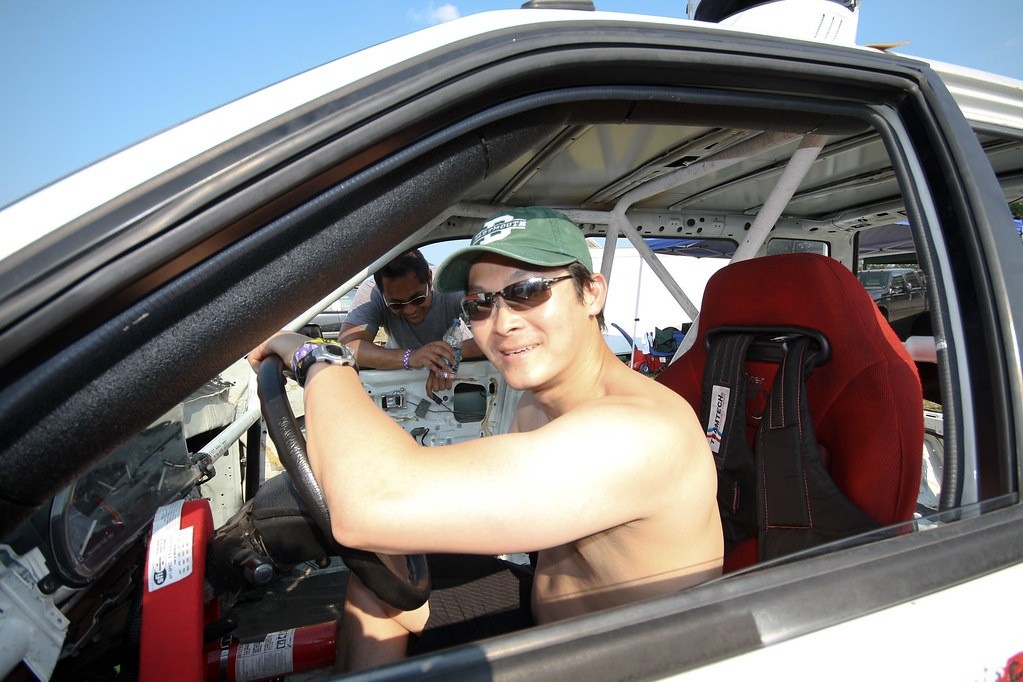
334 247 491 393
245 196 730 671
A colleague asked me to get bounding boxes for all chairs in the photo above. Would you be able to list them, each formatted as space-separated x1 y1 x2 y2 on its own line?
654 252 926 584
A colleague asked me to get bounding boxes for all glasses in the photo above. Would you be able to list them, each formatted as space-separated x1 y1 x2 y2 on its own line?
461 273 571 322
381 283 429 311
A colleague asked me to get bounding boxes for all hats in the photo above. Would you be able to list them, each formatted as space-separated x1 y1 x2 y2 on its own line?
437 206 591 296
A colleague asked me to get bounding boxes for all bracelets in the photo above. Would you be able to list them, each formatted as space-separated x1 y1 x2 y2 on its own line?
403 348 413 370
290 337 355 374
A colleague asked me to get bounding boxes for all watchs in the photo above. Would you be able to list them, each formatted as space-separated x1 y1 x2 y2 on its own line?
292 341 368 386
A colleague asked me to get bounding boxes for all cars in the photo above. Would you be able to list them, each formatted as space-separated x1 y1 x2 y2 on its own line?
0 0 1023 682
